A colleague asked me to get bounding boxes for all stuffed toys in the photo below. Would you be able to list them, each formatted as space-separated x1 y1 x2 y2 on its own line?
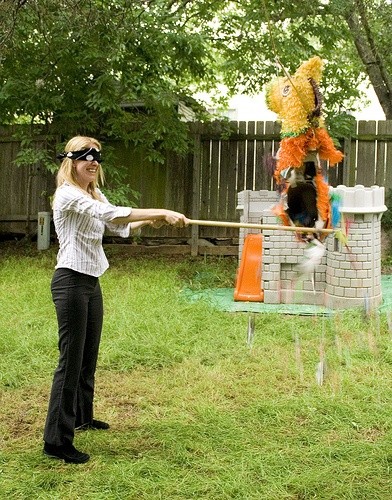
266 56 343 274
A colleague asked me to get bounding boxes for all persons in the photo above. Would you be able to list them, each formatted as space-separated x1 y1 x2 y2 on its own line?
42 136 189 463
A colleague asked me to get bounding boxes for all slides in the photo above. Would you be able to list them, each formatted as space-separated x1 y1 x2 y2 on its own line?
233 232 265 302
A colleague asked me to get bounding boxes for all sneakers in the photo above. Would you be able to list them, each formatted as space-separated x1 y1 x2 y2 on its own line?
76 419 109 429
44 441 90 464
